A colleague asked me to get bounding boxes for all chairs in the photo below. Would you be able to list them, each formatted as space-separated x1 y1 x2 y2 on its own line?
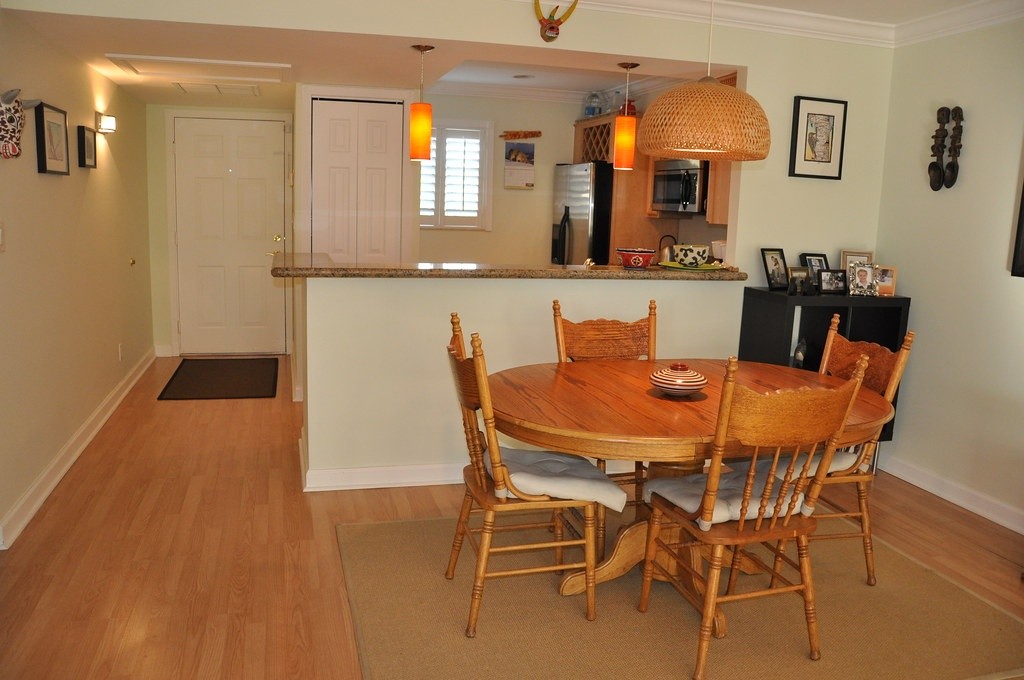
724 311 914 588
547 300 658 560
445 312 624 637
637 354 869 680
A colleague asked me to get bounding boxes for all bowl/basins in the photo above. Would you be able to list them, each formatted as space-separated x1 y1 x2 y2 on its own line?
672 244 710 268
650 363 709 398
615 247 656 270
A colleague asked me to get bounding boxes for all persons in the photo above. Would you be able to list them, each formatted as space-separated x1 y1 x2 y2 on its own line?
770 255 888 290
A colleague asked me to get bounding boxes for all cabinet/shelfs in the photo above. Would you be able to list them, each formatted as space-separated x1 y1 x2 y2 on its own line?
572 108 678 268
311 96 403 269
706 70 737 225
739 286 911 441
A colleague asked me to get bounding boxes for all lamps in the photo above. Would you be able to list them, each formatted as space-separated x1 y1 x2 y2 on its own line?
788 96 848 179
634 0 771 159
409 46 433 159
99 115 117 132
613 62 638 170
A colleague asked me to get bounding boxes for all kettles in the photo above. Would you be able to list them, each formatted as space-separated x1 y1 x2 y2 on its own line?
659 234 676 262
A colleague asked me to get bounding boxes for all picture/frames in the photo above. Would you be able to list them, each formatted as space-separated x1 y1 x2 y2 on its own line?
36 102 70 175
77 125 96 167
761 246 897 296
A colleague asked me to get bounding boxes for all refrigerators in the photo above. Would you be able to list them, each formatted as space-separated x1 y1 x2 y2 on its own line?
551 160 613 265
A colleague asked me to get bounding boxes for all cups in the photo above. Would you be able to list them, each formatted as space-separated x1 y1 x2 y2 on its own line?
711 240 727 263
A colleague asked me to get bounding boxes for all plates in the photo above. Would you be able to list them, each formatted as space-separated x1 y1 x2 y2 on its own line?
658 262 727 272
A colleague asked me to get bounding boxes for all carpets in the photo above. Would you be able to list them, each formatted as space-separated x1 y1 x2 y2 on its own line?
333 505 1024 680
156 358 278 399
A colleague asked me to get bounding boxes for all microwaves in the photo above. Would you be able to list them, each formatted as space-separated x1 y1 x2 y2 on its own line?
651 158 709 214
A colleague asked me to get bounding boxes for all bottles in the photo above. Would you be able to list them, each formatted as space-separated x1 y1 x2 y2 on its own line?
609 91 625 112
619 99 636 115
584 91 602 117
